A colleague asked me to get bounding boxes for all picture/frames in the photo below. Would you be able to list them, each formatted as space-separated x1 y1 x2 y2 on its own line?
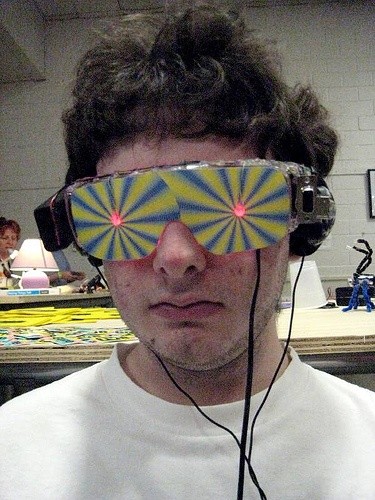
368 169 375 218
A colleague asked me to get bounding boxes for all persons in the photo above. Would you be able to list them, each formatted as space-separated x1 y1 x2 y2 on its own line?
0 217 86 289
0 3 374 500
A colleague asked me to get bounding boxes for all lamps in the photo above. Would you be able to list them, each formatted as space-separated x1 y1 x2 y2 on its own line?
10 239 59 289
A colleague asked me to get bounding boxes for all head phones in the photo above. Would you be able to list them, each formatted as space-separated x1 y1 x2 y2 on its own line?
33 158 336 259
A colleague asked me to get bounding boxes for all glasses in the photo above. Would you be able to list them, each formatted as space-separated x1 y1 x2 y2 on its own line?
63 160 317 262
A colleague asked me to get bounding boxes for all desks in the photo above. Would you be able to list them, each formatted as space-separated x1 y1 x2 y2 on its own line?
0 306 375 398
0 288 114 311
